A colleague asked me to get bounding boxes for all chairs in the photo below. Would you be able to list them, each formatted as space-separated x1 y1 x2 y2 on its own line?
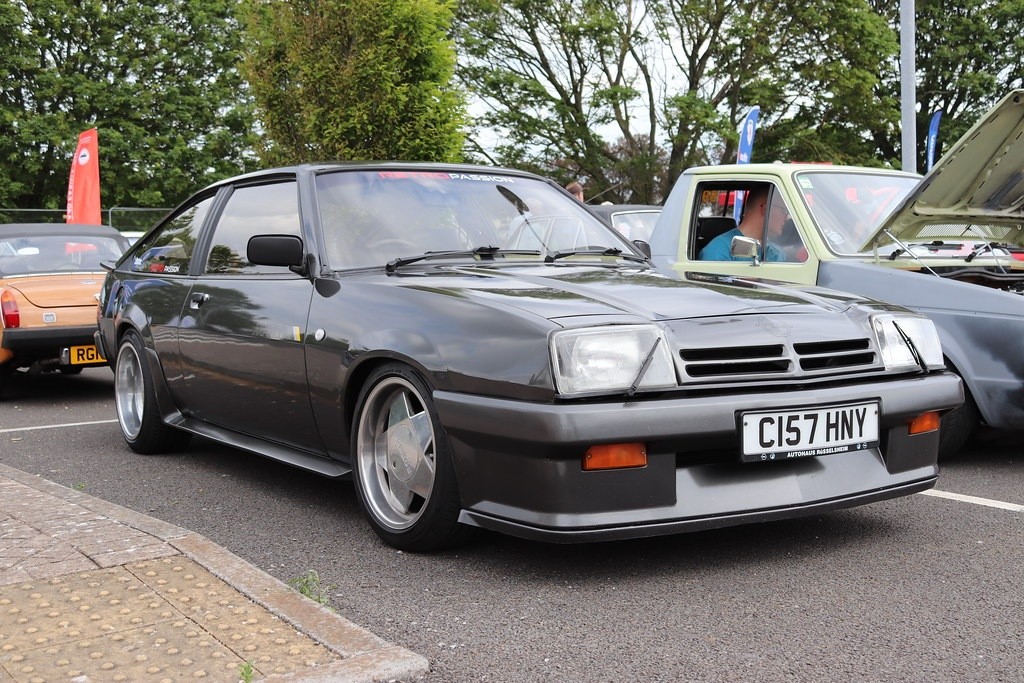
695 217 737 261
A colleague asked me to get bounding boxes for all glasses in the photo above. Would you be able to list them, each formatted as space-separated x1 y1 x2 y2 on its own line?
760 201 790 216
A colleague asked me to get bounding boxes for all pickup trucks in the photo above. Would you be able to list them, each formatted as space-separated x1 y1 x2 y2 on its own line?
642 88 1024 463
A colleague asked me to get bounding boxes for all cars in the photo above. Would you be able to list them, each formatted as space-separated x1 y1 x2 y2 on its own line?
0 221 189 380
93 159 966 547
502 204 667 251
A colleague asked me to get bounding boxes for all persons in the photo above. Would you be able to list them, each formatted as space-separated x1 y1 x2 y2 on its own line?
697 186 791 262
566 182 590 202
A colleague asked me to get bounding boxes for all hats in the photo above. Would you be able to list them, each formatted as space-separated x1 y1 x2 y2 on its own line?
564 181 589 195
